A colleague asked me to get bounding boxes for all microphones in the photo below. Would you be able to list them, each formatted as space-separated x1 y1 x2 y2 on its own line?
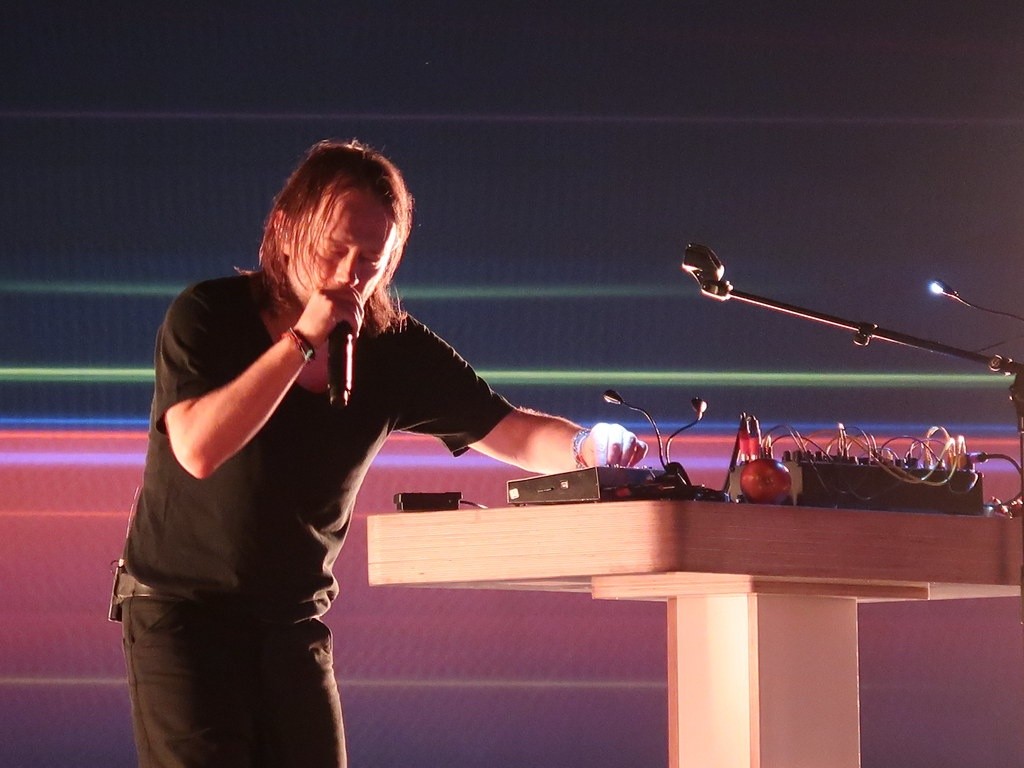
327 320 355 407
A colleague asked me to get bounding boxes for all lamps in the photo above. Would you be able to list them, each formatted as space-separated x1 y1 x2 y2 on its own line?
928 279 1024 321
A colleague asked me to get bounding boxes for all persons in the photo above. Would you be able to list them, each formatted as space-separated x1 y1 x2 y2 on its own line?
106 143 648 768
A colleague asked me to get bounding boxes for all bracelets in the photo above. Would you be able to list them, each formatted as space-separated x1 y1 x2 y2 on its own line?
572 428 592 470
282 326 316 363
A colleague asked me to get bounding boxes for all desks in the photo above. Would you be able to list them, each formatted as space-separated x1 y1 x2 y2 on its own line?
367 499 1024 768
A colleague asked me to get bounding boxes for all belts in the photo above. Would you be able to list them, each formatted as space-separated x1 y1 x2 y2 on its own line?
116 576 176 604
